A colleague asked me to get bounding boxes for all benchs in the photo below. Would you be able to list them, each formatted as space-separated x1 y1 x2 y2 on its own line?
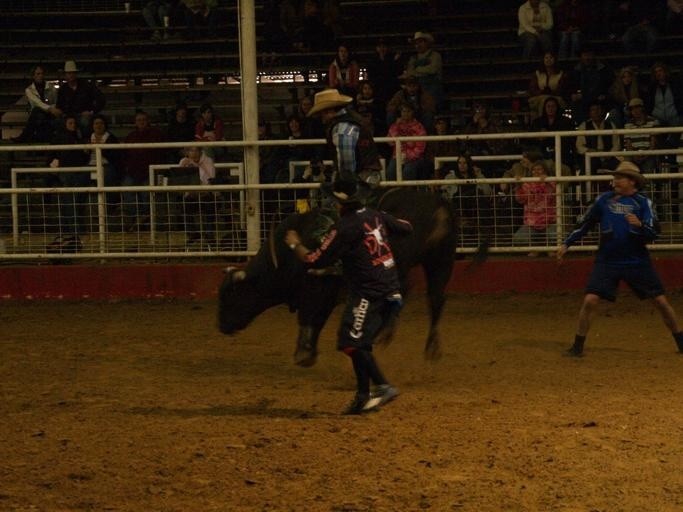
2 0 683 185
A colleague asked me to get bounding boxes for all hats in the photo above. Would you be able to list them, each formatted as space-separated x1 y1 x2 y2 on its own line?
407 31 434 43
305 89 353 118
63 61 79 73
320 169 372 203
598 161 645 184
628 98 642 109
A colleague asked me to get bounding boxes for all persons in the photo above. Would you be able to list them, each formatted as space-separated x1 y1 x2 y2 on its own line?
428 115 457 156
406 32 442 104
275 116 315 182
501 146 571 191
306 90 382 209
263 4 333 66
25 65 61 140
624 17 656 51
573 48 612 113
292 157 331 199
56 61 106 144
166 102 196 163
528 50 570 115
576 100 620 175
194 104 226 156
440 154 493 225
385 75 434 135
552 18 586 56
84 117 121 214
517 1 554 56
386 104 433 180
609 67 639 122
352 82 386 135
367 39 403 83
645 63 681 162
296 96 324 136
558 161 683 357
329 42 359 94
126 112 160 216
461 101 496 155
529 98 570 151
623 97 662 198
178 141 215 242
283 180 414 415
142 3 172 42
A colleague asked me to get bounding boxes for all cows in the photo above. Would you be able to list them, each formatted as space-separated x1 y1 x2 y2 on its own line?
216 187 494 367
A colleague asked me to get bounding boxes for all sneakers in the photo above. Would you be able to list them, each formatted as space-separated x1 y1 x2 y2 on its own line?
561 344 584 359
341 382 399 415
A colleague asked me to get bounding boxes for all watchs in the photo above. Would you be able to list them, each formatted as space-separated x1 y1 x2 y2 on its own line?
290 241 301 250
